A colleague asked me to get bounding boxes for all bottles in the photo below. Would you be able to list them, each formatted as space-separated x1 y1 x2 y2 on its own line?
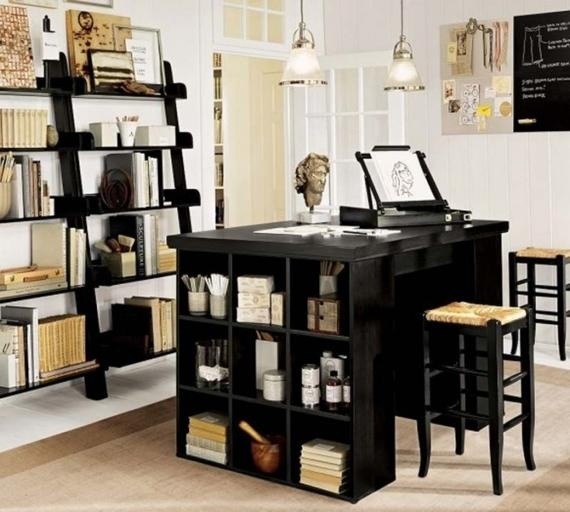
320 352 343 395
343 374 353 415
300 363 320 409
263 369 285 402
325 370 342 413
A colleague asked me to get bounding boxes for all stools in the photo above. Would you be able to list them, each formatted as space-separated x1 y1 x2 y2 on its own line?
415 300 536 496
508 247 570 361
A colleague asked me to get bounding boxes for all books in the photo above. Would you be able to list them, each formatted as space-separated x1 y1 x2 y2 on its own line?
103 149 166 210
1 108 48 149
1 305 42 390
30 220 86 287
11 152 59 220
192 338 228 390
156 245 176 273
186 409 229 466
37 313 98 381
110 210 164 278
1 266 68 297
111 295 178 356
300 437 353 496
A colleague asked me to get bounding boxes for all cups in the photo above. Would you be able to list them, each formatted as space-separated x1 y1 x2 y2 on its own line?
195 344 220 390
117 121 138 146
213 339 228 385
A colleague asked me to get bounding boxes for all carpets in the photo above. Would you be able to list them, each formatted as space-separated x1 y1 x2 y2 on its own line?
0 360 569 511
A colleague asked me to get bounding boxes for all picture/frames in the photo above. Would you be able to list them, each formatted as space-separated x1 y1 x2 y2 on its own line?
112 22 166 96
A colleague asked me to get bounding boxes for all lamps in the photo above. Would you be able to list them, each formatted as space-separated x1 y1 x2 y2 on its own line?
277 0 328 88
383 0 425 91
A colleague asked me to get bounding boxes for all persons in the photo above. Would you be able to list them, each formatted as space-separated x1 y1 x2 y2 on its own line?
445 84 454 98
391 161 415 196
452 100 460 112
296 153 332 208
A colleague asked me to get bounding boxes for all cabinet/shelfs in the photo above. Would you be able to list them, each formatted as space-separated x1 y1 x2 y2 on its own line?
1 51 201 400
175 247 397 505
213 52 223 228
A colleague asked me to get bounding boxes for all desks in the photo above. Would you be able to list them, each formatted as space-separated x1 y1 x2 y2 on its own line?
166 216 509 432
